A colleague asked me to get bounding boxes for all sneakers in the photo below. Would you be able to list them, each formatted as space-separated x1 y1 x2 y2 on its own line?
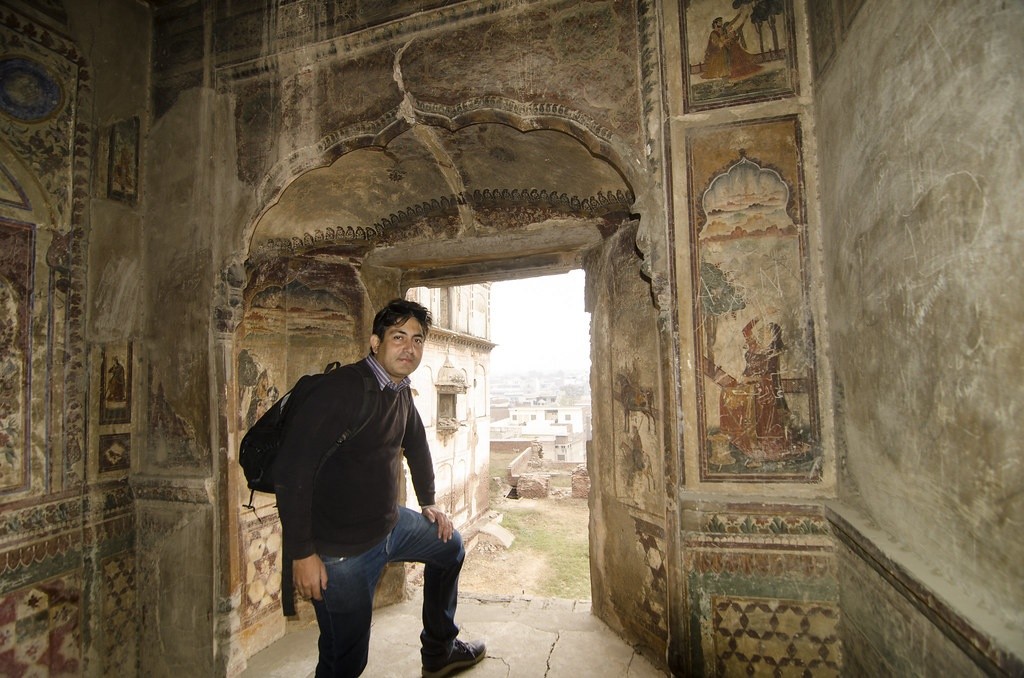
421 638 487 677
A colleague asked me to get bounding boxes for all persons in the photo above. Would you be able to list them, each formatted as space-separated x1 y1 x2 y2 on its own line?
274 298 487 678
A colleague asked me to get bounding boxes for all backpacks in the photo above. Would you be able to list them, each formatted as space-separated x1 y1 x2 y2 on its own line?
239 360 343 523
